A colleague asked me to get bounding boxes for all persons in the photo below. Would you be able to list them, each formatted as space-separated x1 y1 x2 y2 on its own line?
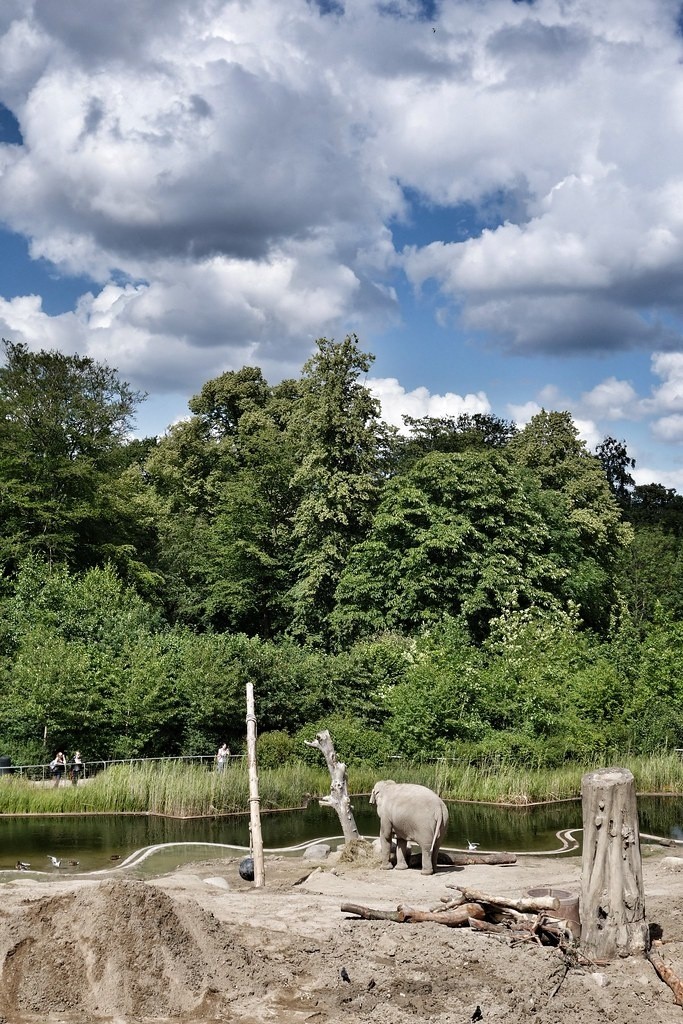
71 752 84 785
217 743 229 774
52 752 66 786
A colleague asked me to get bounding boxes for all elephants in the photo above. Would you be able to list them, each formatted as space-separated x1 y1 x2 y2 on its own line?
367 780 449 875
